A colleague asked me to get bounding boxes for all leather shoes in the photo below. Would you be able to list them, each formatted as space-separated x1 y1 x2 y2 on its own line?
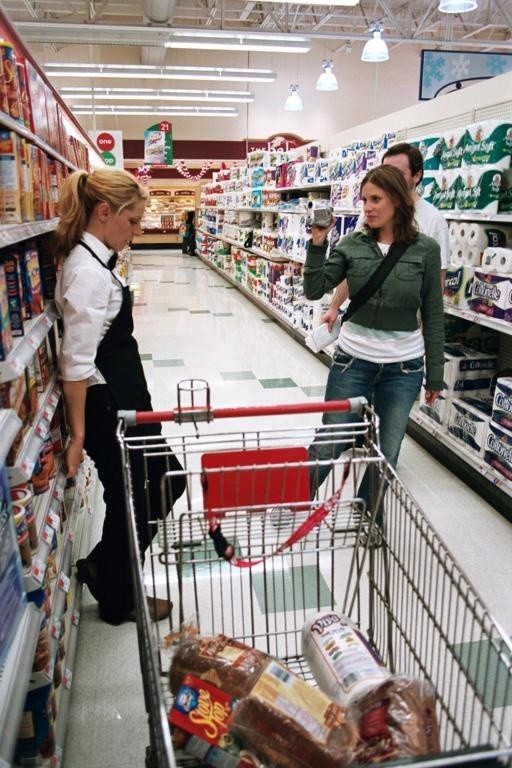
73 557 174 625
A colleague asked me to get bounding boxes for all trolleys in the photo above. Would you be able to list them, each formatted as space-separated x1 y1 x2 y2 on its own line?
112 379 512 766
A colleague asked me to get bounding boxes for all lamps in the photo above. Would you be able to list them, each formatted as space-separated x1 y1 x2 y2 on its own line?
284 1 478 113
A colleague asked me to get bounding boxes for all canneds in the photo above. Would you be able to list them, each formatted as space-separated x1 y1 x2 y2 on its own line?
300 610 392 707
0 412 78 768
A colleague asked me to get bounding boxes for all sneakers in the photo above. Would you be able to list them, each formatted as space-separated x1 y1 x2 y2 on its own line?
350 507 385 549
268 502 298 530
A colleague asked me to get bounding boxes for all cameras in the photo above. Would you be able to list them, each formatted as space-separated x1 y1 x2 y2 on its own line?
306 200 334 228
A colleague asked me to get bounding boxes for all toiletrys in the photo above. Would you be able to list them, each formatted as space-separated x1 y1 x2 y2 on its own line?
271 118 512 486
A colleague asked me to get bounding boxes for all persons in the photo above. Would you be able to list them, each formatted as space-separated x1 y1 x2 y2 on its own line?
320 142 451 453
269 163 444 550
54 171 187 627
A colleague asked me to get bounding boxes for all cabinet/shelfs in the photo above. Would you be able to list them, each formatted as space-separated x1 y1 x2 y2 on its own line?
1 8 109 768
192 154 276 321
276 102 511 522
